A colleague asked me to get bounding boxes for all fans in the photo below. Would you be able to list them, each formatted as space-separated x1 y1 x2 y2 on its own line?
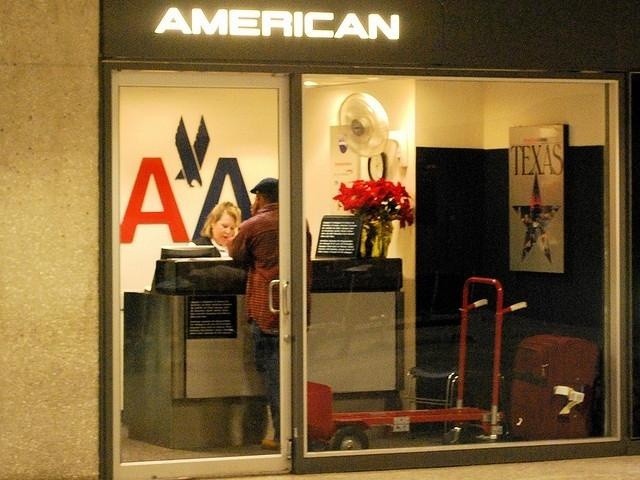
338 90 407 166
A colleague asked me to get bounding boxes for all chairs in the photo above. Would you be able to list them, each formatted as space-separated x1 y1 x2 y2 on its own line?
403 307 497 444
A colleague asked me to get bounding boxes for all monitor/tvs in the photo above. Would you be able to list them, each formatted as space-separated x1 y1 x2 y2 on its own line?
161 247 218 257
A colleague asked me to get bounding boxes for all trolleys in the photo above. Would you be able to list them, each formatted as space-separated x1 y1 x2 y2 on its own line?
305 276 528 453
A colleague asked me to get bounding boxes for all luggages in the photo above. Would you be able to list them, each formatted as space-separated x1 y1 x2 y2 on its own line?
510 334 600 440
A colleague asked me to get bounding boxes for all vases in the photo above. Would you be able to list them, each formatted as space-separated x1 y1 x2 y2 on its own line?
357 216 395 260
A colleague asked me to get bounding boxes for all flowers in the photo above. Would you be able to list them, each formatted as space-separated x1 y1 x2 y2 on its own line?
335 179 415 259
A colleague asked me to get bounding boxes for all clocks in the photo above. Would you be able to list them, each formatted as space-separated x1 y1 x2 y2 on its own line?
367 152 387 181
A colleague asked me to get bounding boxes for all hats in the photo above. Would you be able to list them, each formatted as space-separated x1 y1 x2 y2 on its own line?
251 178 278 194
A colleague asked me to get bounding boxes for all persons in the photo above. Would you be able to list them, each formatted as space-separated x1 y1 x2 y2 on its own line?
227 177 312 449
193 201 241 259
